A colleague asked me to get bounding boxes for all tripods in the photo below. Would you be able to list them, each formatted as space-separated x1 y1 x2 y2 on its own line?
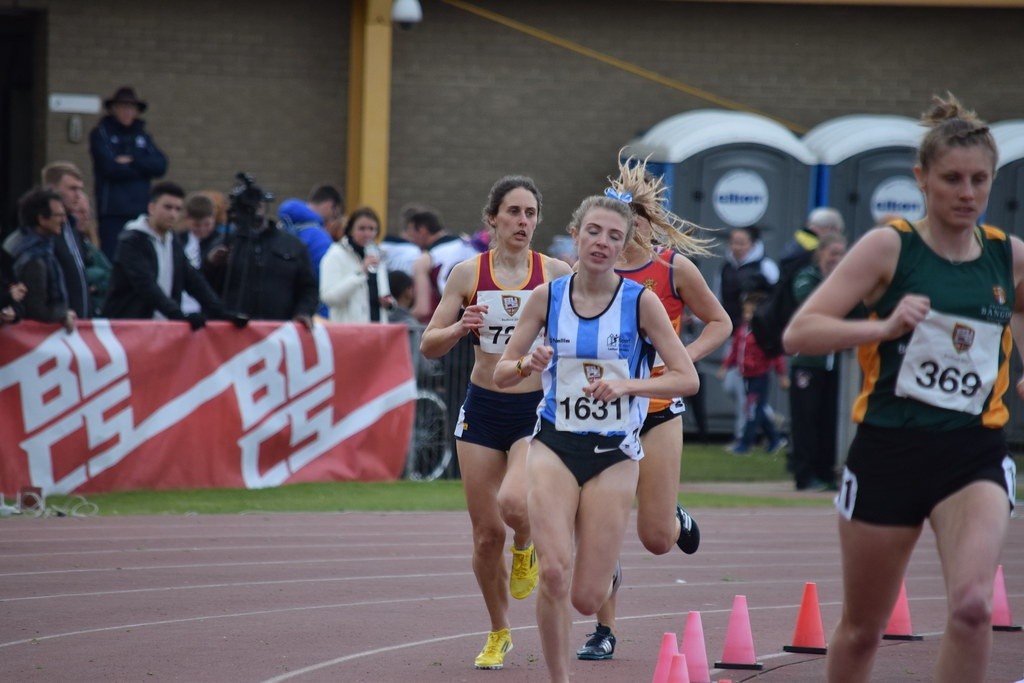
223 208 265 320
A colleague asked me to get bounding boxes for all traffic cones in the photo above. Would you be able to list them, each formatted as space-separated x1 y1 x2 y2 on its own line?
993 565 1022 631
652 632 679 683
678 611 710 683
666 655 690 683
882 580 925 641
714 595 764 669
782 582 827 654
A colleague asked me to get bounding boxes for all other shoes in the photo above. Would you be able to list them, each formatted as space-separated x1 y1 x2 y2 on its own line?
727 443 754 459
767 439 787 453
772 413 784 428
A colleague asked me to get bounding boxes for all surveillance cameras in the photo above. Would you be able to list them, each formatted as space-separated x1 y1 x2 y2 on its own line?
391 0 423 32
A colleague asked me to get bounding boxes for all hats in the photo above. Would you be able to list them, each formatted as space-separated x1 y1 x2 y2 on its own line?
103 87 148 112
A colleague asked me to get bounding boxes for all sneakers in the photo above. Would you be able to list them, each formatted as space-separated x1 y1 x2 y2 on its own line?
508 540 539 599
609 556 622 597
474 628 514 670
577 622 615 659
676 503 701 555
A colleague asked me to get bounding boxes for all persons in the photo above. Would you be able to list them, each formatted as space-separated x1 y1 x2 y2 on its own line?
492 145 701 683
783 90 1024 683
576 165 734 662
419 172 575 670
0 160 495 333
89 85 169 262
717 206 882 492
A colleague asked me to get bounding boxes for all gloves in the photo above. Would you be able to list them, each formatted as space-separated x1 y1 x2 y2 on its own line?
233 314 250 327
187 313 206 330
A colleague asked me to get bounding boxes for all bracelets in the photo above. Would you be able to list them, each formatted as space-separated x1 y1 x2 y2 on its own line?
516 355 532 378
